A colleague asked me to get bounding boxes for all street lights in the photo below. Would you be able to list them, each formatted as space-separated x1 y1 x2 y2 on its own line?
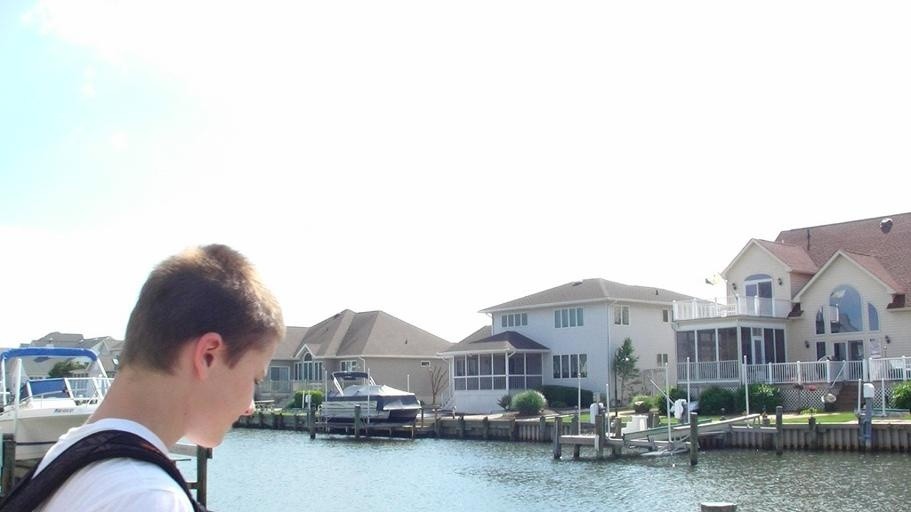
613 356 631 417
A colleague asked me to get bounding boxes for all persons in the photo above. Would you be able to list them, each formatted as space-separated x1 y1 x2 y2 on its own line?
822 392 841 412
671 397 689 426
827 357 832 362
0 241 289 510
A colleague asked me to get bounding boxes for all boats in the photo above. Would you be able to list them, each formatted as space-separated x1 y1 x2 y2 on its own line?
0 347 118 478
317 368 424 421
